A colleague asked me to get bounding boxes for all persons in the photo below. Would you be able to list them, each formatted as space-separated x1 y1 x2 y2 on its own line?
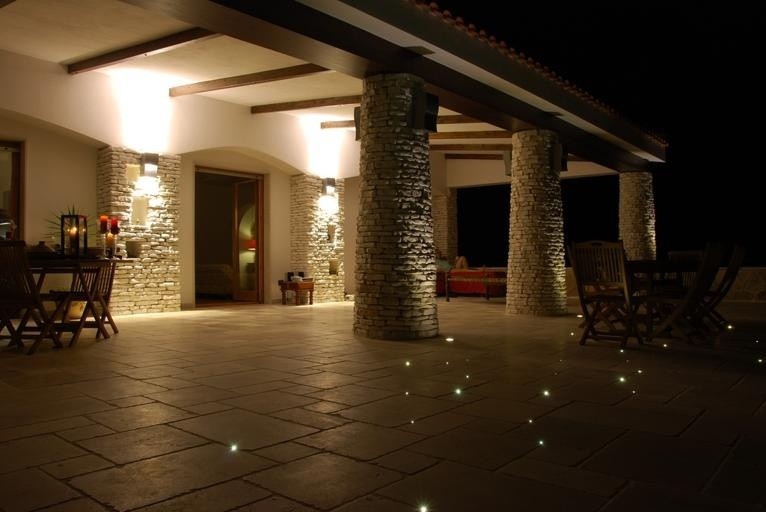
434 248 468 274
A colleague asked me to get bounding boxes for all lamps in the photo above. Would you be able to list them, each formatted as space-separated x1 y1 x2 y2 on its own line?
139 152 159 176
408 92 439 134
503 151 512 176
354 106 361 142
548 144 569 178
321 178 337 195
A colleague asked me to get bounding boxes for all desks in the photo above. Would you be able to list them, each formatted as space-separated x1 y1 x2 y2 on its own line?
278 279 314 306
7 258 135 357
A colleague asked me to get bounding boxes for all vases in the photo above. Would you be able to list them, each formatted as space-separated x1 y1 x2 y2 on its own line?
126 239 144 258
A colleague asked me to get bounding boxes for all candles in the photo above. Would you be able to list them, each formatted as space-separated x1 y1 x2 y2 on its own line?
110 215 119 234
100 214 108 233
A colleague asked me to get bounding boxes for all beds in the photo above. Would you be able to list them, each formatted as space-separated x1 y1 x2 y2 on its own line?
436 267 506 297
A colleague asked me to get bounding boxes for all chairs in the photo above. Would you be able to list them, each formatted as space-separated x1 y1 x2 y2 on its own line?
0 239 119 355
565 240 746 350
